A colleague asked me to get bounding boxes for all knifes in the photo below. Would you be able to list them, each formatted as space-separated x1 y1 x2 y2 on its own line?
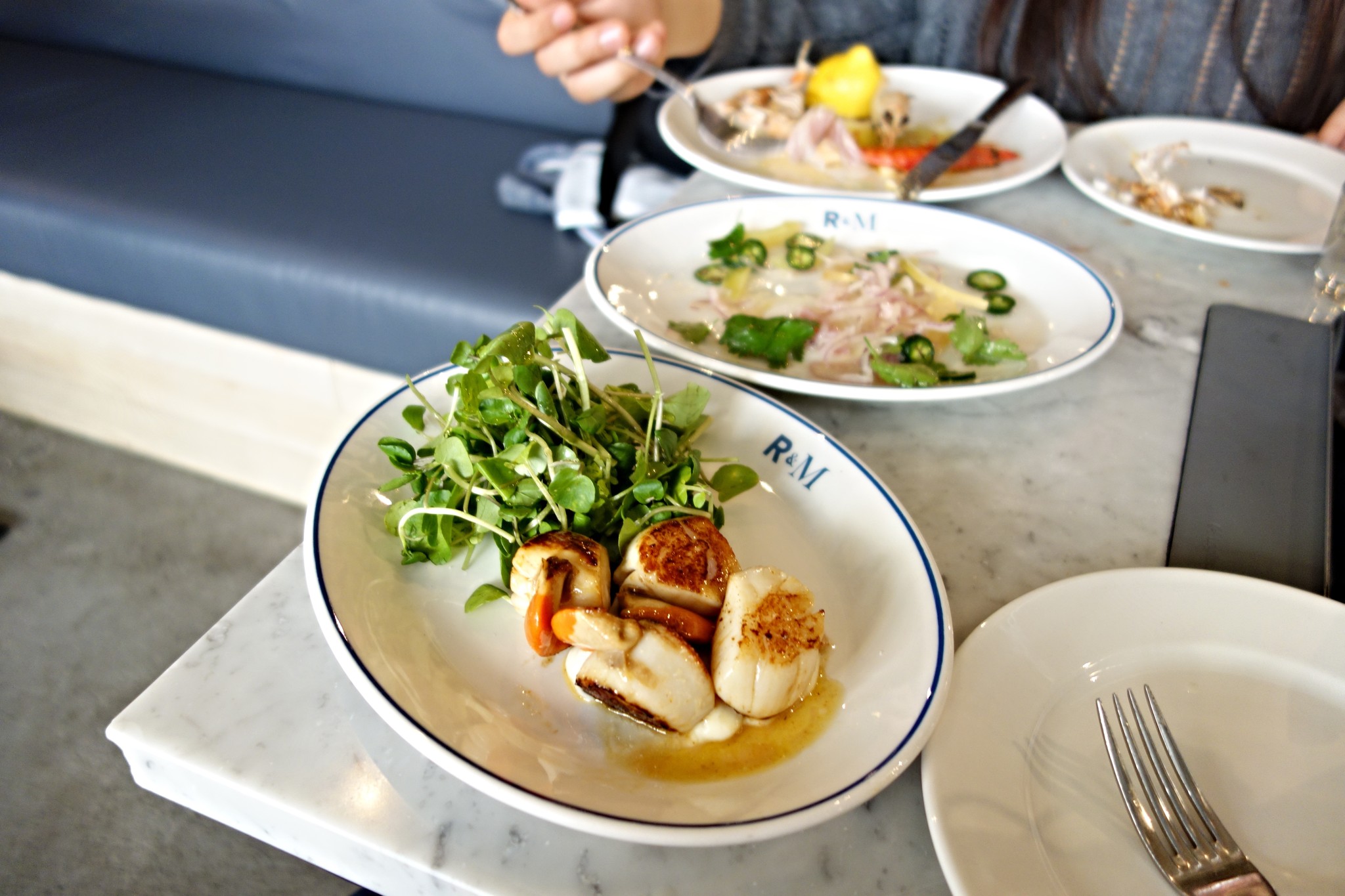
899 71 1037 200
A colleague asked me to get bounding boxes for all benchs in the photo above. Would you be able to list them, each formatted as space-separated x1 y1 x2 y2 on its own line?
3 0 649 528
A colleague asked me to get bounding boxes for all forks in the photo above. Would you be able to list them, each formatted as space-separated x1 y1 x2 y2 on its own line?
1094 680 1279 895
506 1 790 160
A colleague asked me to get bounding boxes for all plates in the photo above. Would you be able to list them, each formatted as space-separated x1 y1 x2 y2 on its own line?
300 344 956 846
920 566 1345 895
583 192 1125 402
1059 112 1345 256
654 66 1068 203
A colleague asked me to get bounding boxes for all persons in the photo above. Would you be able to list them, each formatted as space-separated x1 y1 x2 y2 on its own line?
490 0 1345 199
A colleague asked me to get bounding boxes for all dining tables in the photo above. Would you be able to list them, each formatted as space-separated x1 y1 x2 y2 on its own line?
90 157 1345 896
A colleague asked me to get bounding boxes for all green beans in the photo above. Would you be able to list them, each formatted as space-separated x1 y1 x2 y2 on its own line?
338 309 762 583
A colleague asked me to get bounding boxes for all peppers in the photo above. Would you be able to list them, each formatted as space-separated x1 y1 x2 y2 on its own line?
672 225 1020 396
